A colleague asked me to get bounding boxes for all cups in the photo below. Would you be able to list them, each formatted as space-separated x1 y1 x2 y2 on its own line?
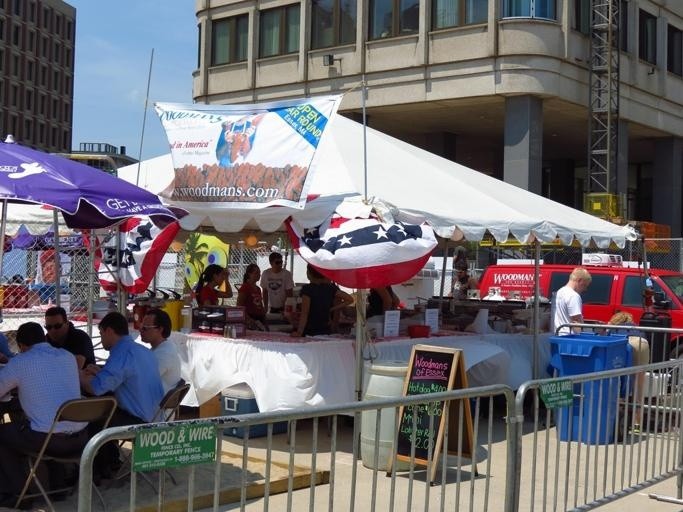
493 318 511 333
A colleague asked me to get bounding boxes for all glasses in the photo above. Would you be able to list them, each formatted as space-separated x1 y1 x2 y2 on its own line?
140 323 158 331
44 322 65 330
273 260 282 265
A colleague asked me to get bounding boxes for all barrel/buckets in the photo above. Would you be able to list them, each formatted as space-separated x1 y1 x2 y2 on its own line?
359 359 411 470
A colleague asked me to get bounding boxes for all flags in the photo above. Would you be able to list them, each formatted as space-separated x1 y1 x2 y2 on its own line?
149 101 339 211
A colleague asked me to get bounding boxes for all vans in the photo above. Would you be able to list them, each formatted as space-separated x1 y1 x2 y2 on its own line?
469 257 681 397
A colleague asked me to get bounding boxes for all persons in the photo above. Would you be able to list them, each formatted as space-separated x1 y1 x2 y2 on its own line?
363 281 399 321
0 304 183 511
288 264 352 336
445 255 477 303
258 252 292 316
551 267 592 336
192 265 232 307
604 310 650 435
235 263 267 331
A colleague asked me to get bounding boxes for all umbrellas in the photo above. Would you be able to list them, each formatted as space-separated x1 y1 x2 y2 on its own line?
0 133 190 286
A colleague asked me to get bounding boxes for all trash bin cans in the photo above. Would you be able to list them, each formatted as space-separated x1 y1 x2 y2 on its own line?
548 332 634 445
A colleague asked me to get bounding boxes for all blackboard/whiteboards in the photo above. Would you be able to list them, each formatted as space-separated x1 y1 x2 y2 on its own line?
442 348 474 458
394 345 461 467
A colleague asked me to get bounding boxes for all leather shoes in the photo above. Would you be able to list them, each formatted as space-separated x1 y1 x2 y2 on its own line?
0 461 122 510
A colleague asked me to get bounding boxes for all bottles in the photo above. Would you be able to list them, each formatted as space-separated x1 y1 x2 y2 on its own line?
137 297 162 307
180 305 192 333
224 325 236 338
283 297 302 318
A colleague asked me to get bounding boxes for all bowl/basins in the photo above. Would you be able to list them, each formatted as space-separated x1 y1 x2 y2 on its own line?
409 324 431 338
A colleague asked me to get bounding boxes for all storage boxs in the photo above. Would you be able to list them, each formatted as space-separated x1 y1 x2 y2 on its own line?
219 385 301 439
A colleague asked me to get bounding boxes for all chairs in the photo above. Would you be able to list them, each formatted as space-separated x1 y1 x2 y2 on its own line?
11 395 117 511
111 383 190 494
